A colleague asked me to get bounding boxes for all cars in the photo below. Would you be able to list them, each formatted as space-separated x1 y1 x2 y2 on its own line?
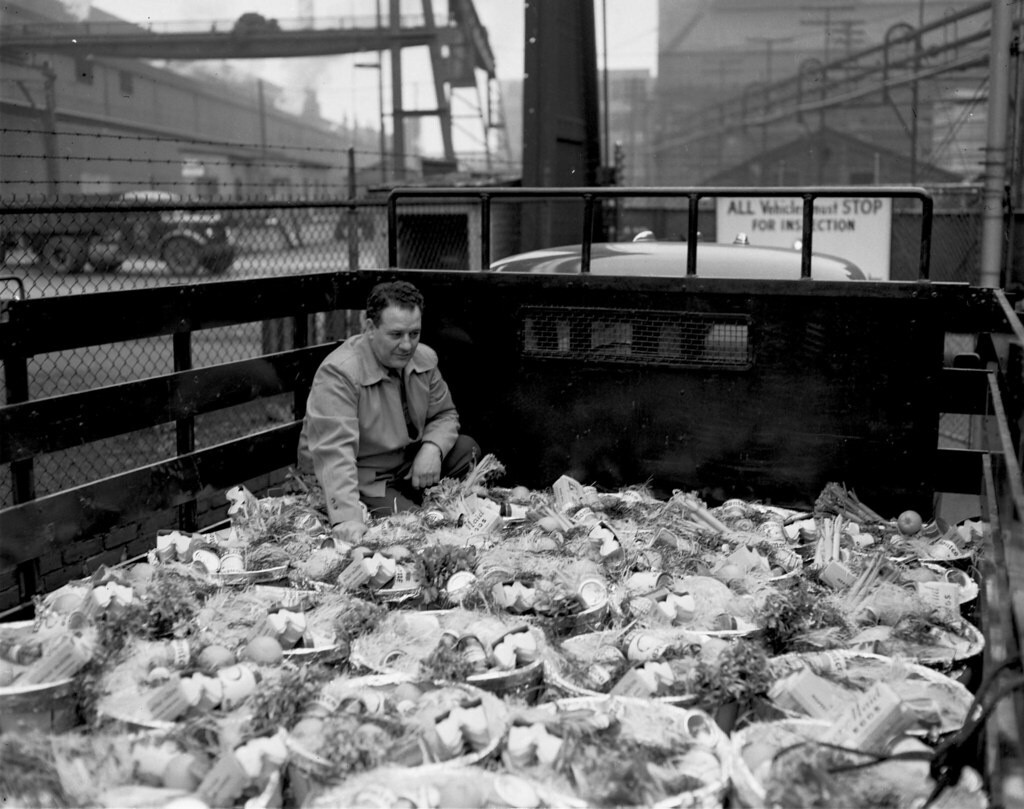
42 189 234 277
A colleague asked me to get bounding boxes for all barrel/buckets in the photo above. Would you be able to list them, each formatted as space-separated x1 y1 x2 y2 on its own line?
0 543 984 809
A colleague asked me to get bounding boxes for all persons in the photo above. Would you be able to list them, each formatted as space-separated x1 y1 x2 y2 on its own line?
295 280 481 531
